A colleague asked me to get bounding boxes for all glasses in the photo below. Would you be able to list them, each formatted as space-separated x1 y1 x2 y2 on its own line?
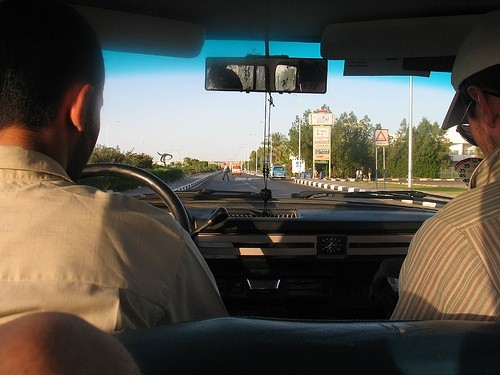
456 99 479 148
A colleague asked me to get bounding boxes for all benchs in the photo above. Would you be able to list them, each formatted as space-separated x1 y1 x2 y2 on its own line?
109 316 500 375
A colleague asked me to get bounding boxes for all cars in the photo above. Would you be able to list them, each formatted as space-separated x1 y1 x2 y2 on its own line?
269 165 286 180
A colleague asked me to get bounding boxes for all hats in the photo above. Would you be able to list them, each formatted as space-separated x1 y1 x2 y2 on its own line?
437 11 500 130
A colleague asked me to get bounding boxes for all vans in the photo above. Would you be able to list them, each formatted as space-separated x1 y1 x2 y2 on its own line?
232 165 242 175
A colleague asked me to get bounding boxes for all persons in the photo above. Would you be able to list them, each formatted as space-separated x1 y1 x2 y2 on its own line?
308 168 372 180
0 0 224 332
0 310 140 375
222 163 229 182
389 24 500 320
297 64 325 92
205 68 243 90
